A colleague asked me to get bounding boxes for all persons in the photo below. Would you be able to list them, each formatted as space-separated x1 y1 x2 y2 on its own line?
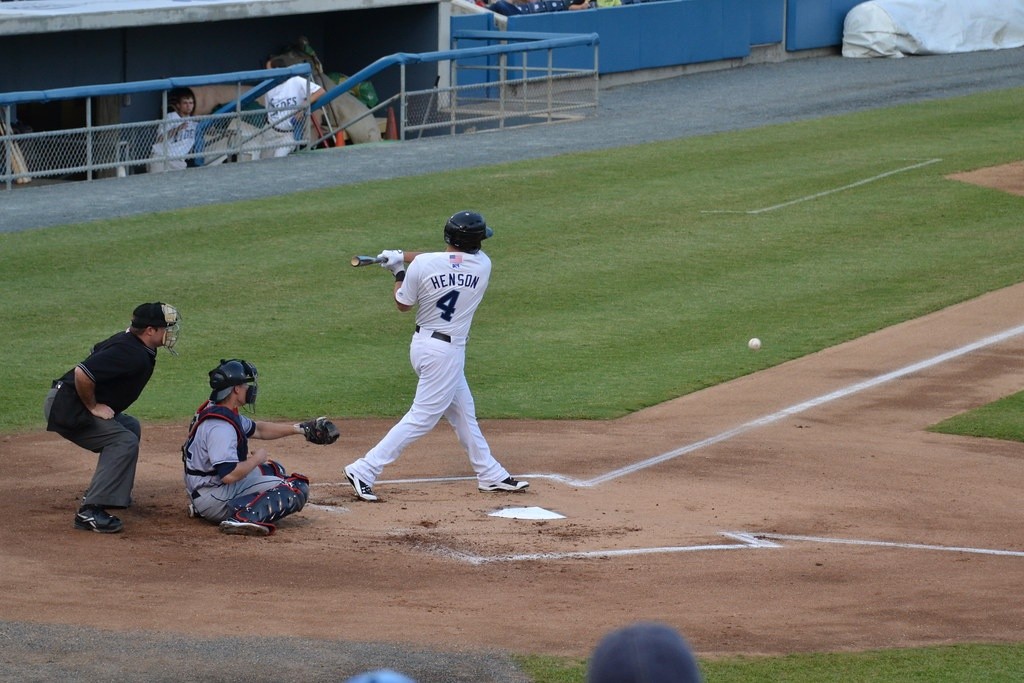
182 356 340 534
260 57 326 161
147 87 216 172
466 0 622 16
341 211 530 501
589 621 700 683
45 301 183 533
348 670 412 683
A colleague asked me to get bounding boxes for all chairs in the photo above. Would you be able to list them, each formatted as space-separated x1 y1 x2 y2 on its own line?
515 0 649 14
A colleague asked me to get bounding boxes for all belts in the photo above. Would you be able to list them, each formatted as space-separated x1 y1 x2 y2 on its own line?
415 324 451 343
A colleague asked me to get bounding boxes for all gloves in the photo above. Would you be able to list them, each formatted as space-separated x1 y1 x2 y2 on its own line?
376 249 405 277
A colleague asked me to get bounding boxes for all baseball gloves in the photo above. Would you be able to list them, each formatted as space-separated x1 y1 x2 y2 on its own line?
300 415 340 446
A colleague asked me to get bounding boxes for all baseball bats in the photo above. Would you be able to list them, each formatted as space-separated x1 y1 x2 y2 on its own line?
350 255 389 267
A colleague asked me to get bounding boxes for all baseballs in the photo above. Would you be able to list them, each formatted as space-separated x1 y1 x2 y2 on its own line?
749 336 762 351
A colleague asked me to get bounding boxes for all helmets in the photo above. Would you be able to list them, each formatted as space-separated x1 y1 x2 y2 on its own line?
131 302 168 328
208 358 257 401
443 210 494 254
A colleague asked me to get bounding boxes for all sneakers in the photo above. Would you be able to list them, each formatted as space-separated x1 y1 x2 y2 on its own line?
344 466 378 498
478 476 529 491
220 516 269 535
74 506 123 532
